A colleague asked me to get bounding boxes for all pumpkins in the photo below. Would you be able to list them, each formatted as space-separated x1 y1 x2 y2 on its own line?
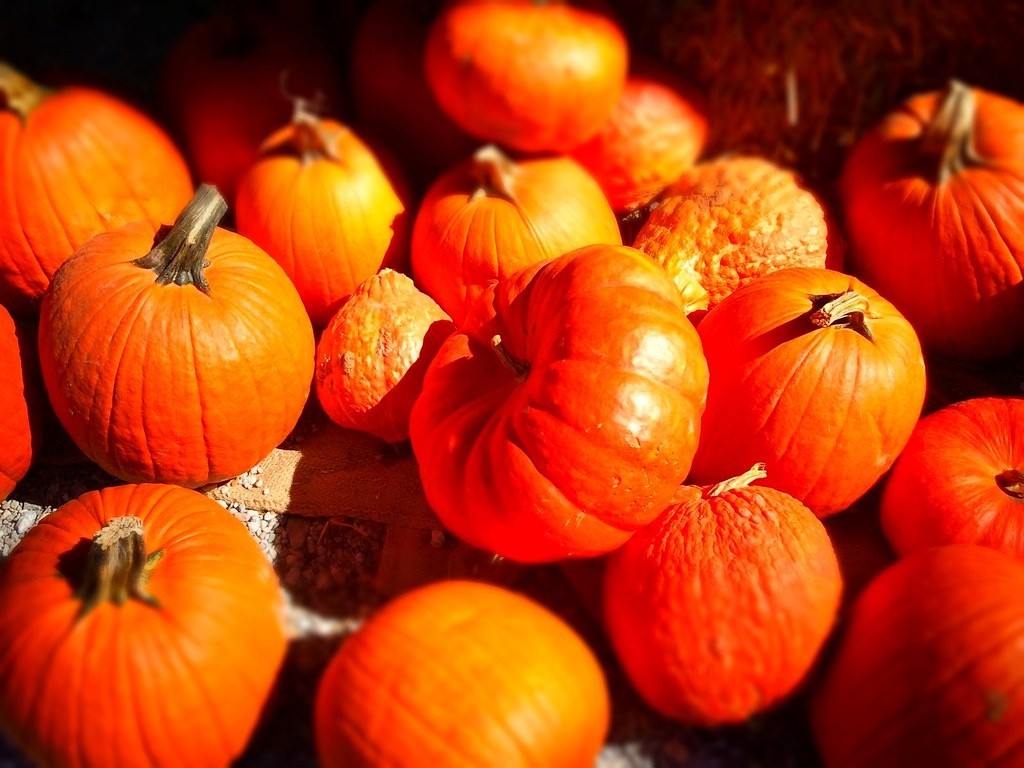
1 0 1024 768
